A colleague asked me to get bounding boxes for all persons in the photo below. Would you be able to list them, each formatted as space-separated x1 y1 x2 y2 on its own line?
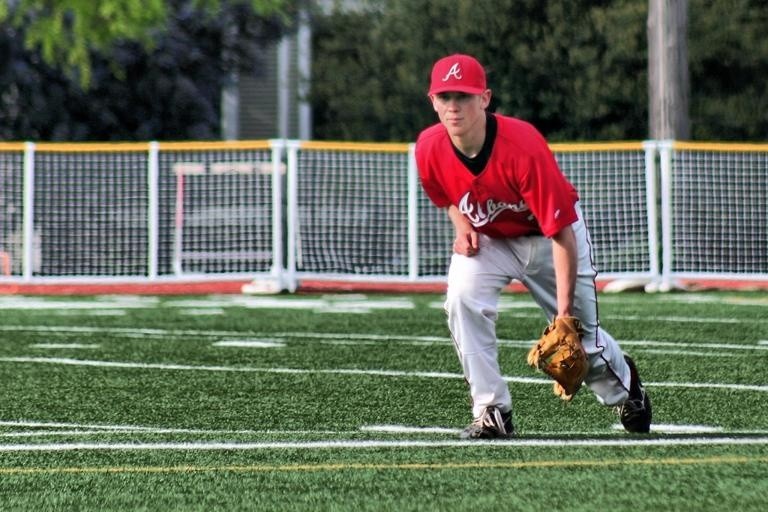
413 52 654 440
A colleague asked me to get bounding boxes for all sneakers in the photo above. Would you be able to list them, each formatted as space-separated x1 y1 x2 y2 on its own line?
461 408 518 444
613 349 653 437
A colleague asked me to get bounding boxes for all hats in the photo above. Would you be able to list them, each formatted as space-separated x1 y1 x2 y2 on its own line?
425 51 488 99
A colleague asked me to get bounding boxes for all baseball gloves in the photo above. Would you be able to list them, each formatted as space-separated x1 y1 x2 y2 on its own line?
527 317 591 403
